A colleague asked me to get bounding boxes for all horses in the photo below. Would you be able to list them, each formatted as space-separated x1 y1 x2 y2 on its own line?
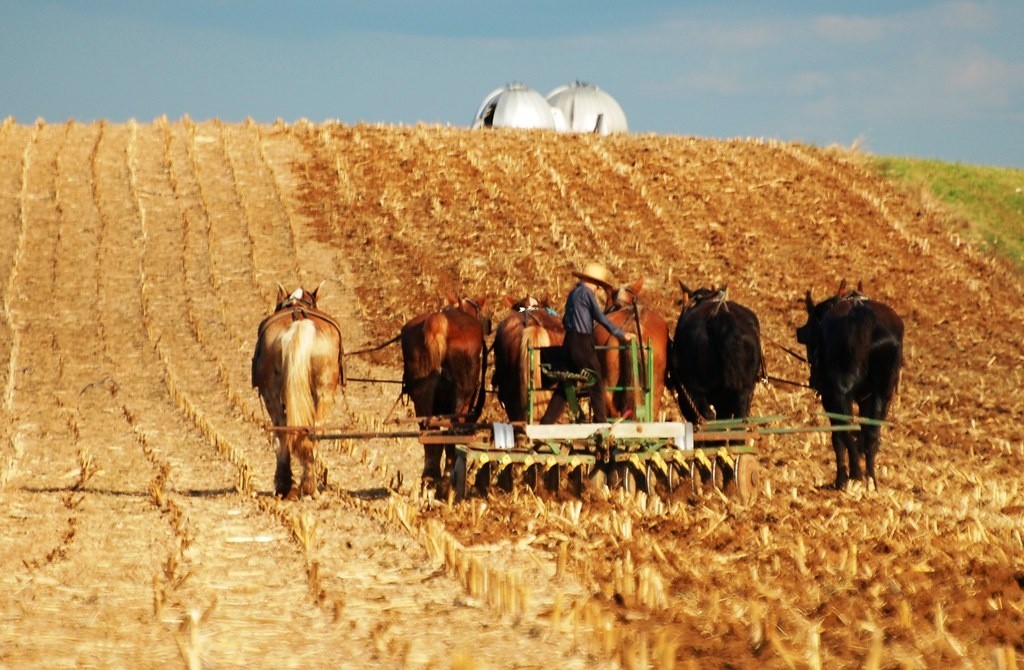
401 290 491 484
595 276 669 421
493 294 567 424
670 280 766 447
797 279 905 492
252 279 342 498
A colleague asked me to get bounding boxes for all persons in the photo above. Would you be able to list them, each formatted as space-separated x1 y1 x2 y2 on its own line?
538 262 637 424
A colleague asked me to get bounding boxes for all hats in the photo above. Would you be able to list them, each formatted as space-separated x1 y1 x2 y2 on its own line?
571 262 613 292
